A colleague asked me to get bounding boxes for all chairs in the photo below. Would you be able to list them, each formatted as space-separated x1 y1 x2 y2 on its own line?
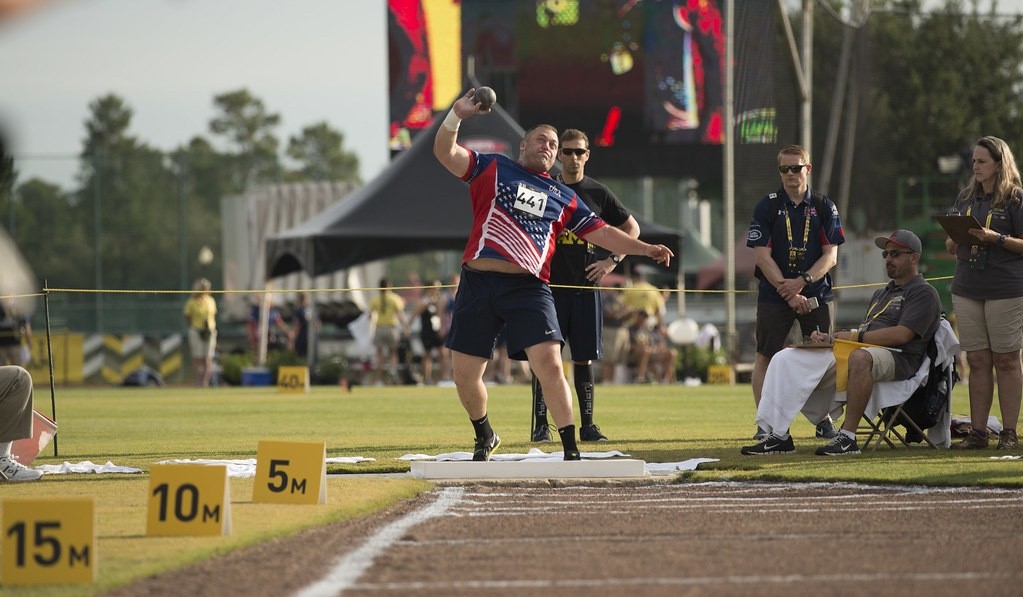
834 315 948 457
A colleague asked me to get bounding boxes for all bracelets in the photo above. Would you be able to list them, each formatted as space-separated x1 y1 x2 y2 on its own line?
858 331 865 342
999 235 1006 247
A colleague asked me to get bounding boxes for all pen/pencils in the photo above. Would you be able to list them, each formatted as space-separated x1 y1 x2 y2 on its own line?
816 325 819 335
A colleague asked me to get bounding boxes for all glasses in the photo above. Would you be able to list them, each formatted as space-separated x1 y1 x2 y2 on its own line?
560 147 588 156
779 164 807 174
882 249 914 258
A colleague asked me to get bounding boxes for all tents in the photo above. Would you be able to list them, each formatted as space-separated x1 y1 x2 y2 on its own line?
259 85 686 367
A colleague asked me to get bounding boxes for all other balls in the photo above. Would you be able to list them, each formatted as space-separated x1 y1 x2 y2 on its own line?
473 86 496 110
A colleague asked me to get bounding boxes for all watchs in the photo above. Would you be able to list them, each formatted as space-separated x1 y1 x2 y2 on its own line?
801 272 812 285
609 254 620 265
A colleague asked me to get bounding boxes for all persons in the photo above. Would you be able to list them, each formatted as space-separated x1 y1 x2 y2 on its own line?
740 229 942 458
244 267 676 387
0 366 45 481
745 145 846 438
529 129 641 443
433 87 675 461
0 291 32 368
182 278 220 388
947 137 1023 450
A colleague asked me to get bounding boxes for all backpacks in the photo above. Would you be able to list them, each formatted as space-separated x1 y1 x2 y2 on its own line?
884 314 961 443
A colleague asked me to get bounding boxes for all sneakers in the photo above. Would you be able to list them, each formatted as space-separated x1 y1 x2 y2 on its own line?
473 429 501 462
996 429 1018 450
741 432 797 454
815 432 862 455
564 450 581 460
580 424 609 442
0 454 45 482
752 425 773 439
950 431 989 450
534 423 551 443
815 417 840 439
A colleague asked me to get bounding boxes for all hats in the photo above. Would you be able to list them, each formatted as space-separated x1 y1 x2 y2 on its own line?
875 230 922 256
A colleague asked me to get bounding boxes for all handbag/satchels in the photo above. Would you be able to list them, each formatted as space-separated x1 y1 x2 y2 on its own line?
951 414 1003 437
